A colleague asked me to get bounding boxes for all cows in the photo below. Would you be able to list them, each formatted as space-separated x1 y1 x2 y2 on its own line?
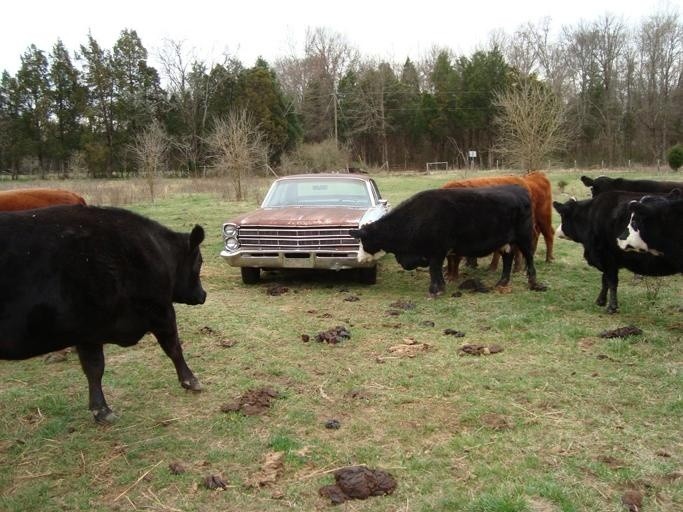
438 171 556 282
0 188 89 364
348 184 538 298
0 203 207 425
552 175 683 315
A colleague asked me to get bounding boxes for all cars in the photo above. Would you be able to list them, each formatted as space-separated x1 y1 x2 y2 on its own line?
219 174 392 284
